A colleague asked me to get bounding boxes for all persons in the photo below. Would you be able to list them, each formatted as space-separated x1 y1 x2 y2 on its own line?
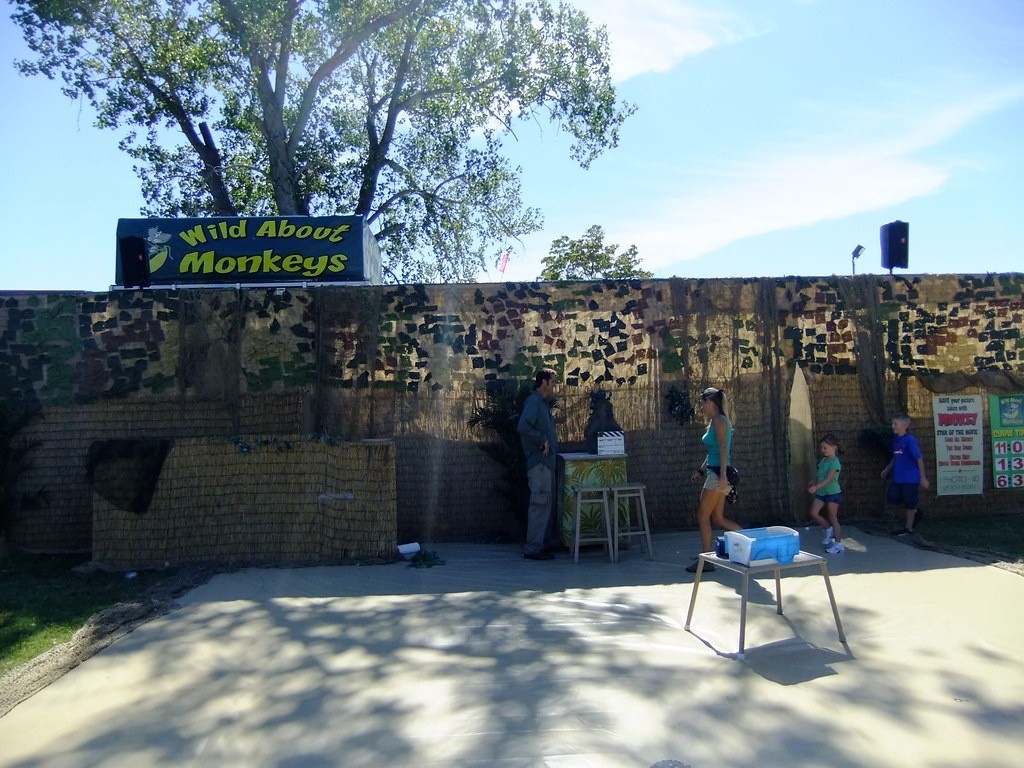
686 387 745 573
881 412 929 536
518 367 570 560
806 435 845 556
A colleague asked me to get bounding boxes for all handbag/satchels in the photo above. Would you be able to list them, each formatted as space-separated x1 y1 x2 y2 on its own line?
707 466 738 481
727 481 739 504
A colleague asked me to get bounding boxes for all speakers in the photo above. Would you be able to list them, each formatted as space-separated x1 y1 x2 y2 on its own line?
118 234 152 288
879 220 909 270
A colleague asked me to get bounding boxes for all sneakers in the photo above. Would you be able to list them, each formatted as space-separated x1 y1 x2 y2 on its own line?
822 526 834 545
825 542 845 554
686 559 714 572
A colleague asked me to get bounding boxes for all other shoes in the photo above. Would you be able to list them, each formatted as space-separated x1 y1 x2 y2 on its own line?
912 509 923 528
896 528 913 536
524 543 550 560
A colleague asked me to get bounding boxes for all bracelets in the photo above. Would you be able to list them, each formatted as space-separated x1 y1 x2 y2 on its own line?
697 469 704 477
816 485 820 489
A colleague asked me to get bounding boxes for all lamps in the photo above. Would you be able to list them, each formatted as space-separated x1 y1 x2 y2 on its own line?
852 244 865 275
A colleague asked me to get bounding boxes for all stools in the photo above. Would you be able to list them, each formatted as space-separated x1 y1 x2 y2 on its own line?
568 481 654 564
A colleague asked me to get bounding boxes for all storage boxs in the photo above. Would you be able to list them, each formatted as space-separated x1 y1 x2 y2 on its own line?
716 526 800 568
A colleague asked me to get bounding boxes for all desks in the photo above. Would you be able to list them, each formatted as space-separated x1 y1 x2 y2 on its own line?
683 548 846 661
557 453 632 551
